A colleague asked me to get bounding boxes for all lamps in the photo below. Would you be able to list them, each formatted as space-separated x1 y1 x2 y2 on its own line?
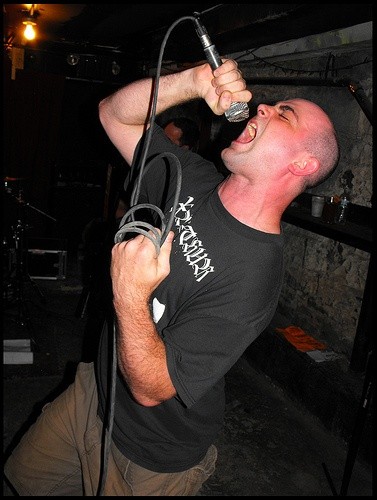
19 4 42 41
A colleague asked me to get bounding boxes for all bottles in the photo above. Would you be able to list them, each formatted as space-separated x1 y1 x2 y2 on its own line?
325 187 349 226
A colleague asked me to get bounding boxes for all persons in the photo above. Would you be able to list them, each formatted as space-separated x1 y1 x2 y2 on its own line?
0 58 341 500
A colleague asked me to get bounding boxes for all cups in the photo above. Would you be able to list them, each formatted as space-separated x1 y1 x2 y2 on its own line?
311 196 324 217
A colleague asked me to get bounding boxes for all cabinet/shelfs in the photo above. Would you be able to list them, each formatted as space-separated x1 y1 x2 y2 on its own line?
197 75 377 498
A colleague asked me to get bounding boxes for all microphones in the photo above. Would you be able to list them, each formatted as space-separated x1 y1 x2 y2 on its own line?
197 26 249 122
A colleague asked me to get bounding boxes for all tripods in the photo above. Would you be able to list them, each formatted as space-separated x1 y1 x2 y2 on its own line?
0 235 48 353
